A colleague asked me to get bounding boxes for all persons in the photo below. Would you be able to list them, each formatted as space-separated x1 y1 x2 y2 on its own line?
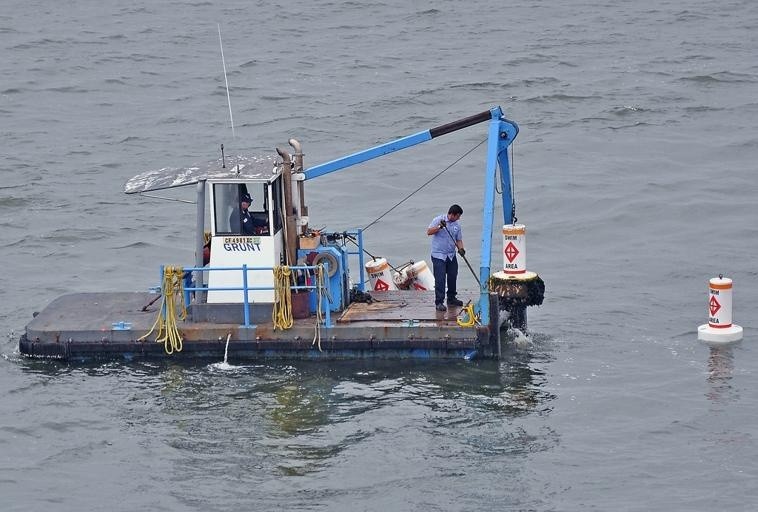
229 192 267 234
426 204 467 311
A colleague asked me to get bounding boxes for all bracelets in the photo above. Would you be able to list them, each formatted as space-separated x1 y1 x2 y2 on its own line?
438 224 440 228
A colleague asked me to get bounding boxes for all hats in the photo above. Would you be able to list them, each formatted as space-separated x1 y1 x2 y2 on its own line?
241 193 253 203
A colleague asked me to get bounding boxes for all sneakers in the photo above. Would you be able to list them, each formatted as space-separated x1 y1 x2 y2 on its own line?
447 297 463 306
436 303 447 311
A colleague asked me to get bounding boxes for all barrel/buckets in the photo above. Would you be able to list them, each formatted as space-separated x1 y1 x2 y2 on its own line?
291 291 310 318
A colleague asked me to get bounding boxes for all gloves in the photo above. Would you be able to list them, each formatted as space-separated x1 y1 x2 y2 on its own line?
458 248 465 257
439 219 447 230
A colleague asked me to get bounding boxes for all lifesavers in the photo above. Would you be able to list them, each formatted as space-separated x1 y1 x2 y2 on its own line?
313 253 338 277
